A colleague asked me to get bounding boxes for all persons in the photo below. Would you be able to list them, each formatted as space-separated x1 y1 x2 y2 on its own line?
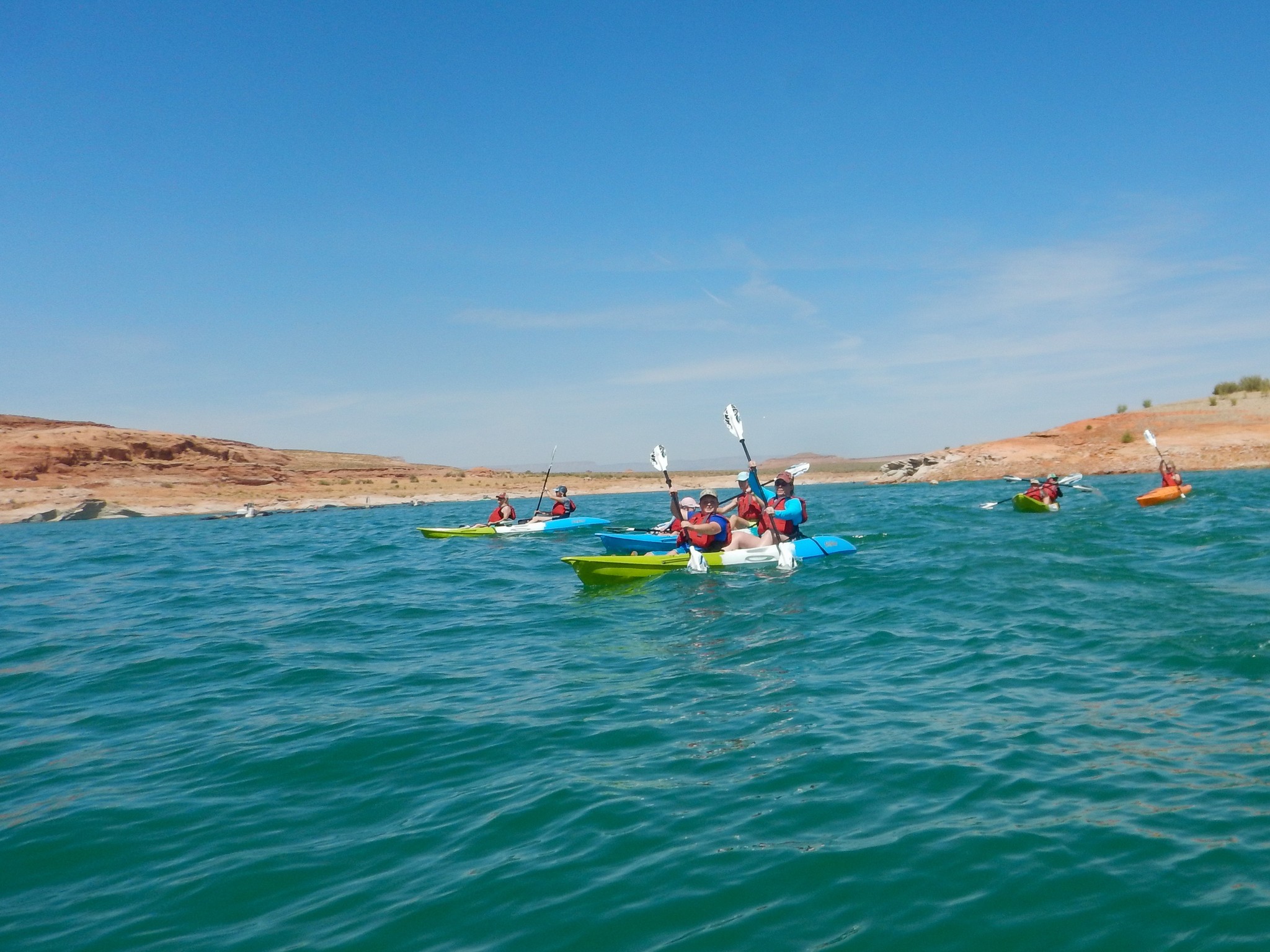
1159 459 1183 487
525 485 570 524
469 492 512 529
643 471 803 556
1023 473 1063 505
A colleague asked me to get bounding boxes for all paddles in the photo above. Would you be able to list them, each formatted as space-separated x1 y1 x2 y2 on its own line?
722 402 800 571
458 518 514 528
602 524 673 535
717 463 811 501
1143 428 1187 498
533 444 557 518
648 443 711 574
1003 474 1098 493
978 474 1085 511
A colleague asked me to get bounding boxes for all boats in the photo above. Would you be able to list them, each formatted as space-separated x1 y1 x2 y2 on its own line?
559 533 858 587
1011 493 1061 513
1135 484 1192 507
594 526 762 553
415 516 612 539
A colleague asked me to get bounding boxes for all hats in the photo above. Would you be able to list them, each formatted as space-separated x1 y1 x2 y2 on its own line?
496 493 508 498
681 497 700 508
1047 474 1058 480
776 471 791 483
700 489 718 499
1031 480 1040 485
735 472 749 482
553 486 567 493
